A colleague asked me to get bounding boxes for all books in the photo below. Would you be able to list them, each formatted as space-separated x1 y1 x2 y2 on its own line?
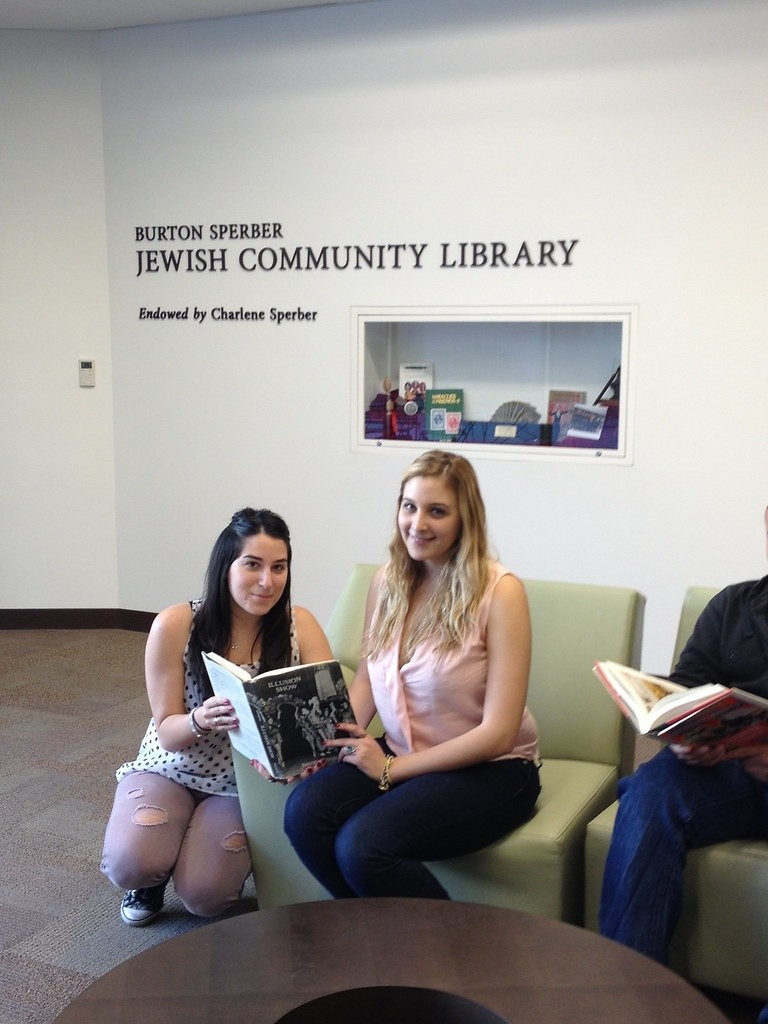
200 649 360 781
593 658 767 753
393 363 617 449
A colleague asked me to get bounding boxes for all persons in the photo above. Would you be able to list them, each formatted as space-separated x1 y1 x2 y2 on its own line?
289 451 545 901
100 508 337 927
601 508 768 971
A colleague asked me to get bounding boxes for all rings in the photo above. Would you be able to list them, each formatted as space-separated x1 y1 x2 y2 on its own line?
344 744 355 754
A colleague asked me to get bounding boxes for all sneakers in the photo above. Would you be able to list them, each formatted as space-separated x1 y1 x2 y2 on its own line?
120 883 164 928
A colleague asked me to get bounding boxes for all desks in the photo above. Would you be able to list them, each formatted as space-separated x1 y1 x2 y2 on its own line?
45 892 737 1023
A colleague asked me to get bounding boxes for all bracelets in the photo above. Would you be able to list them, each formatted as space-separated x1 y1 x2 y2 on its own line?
378 752 396 792
188 706 213 739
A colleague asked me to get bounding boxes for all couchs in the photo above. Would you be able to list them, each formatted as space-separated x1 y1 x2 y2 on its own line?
583 582 766 1005
229 557 644 929
